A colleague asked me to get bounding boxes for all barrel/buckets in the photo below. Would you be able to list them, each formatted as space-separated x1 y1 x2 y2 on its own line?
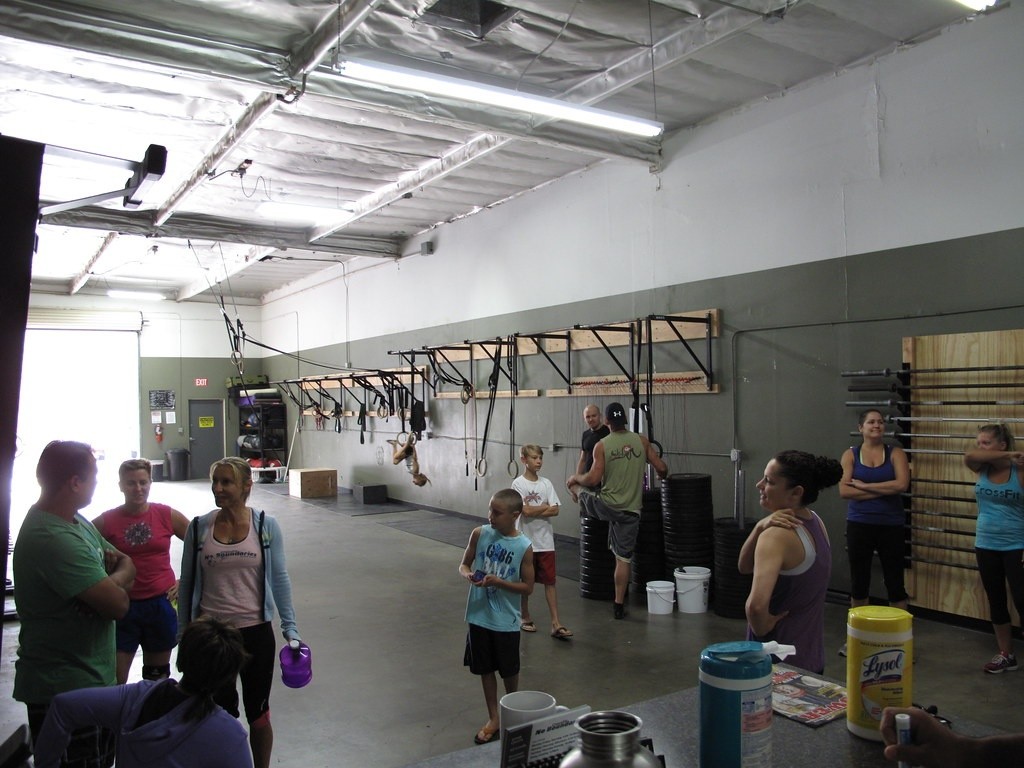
674 566 713 613
646 581 677 615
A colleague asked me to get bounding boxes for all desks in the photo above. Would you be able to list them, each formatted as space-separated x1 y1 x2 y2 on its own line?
394 664 1024 767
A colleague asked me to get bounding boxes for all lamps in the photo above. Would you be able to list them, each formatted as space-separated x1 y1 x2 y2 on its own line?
331 0 664 139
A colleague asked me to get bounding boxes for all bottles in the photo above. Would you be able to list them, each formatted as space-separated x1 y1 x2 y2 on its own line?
558 709 663 768
845 605 914 741
279 640 313 689
697 641 775 768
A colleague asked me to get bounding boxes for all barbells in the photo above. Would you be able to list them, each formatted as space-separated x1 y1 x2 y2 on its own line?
840 365 1024 571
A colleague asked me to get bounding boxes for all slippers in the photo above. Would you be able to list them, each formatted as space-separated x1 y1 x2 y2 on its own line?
520 621 536 631
474 724 500 743
550 626 573 637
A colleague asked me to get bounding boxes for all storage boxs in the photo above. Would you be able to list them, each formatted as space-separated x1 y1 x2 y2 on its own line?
352 484 389 503
289 468 339 498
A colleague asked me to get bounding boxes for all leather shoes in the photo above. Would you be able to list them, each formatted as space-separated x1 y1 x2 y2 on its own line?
614 608 626 619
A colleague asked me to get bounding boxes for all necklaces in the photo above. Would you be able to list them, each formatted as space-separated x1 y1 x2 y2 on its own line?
222 513 233 543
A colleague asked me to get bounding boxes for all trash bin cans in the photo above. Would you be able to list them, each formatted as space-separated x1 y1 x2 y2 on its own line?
165 448 189 482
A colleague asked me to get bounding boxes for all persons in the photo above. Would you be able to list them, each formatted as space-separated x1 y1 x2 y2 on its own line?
577 404 610 488
510 444 573 638
12 440 136 768
566 402 668 619
92 459 191 685
964 423 1024 674
738 449 843 675
386 431 432 487
458 489 535 744
838 409 911 657
174 456 302 768
34 614 253 768
880 706 1024 768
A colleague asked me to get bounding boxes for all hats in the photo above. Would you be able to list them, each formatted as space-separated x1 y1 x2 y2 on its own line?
605 402 627 426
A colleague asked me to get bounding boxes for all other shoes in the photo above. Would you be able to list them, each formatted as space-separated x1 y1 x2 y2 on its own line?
839 642 847 657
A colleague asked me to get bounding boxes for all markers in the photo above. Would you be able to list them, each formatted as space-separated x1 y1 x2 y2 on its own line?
896 713 910 768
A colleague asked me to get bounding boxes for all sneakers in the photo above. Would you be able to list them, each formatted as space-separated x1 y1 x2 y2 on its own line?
984 652 1018 673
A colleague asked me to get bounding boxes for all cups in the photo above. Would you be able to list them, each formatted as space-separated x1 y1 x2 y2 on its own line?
498 690 569 745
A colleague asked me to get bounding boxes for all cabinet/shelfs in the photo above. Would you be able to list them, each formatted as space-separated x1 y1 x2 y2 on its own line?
238 404 288 483
